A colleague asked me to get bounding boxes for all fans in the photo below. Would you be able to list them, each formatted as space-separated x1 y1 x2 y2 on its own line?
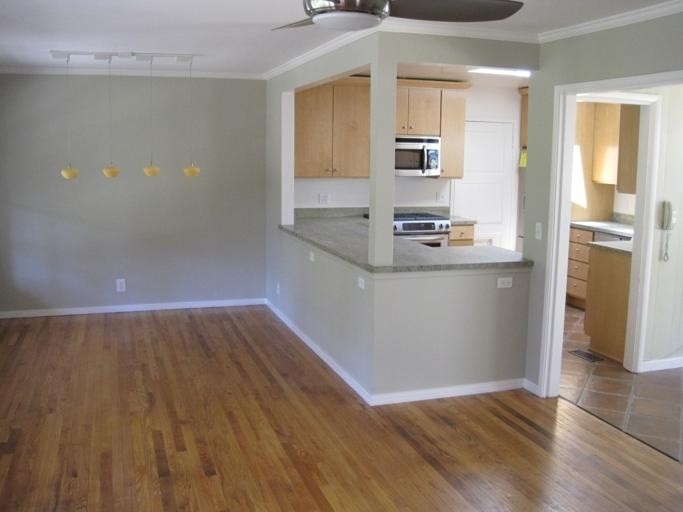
270 0 524 32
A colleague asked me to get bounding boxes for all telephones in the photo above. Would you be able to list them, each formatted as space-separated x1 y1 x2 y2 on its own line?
662 200 677 230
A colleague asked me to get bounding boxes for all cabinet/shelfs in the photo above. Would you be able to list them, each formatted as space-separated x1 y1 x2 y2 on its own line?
395 82 441 136
567 226 594 301
449 225 474 245
294 75 370 177
440 81 465 179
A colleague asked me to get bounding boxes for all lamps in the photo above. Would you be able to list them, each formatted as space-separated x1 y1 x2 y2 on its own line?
49 46 213 181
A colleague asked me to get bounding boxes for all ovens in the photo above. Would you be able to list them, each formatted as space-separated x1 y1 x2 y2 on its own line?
399 233 449 248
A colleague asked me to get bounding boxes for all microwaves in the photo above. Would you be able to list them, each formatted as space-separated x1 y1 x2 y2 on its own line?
394 135 441 178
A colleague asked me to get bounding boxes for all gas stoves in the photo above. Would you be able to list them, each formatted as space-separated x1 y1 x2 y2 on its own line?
363 213 452 236
